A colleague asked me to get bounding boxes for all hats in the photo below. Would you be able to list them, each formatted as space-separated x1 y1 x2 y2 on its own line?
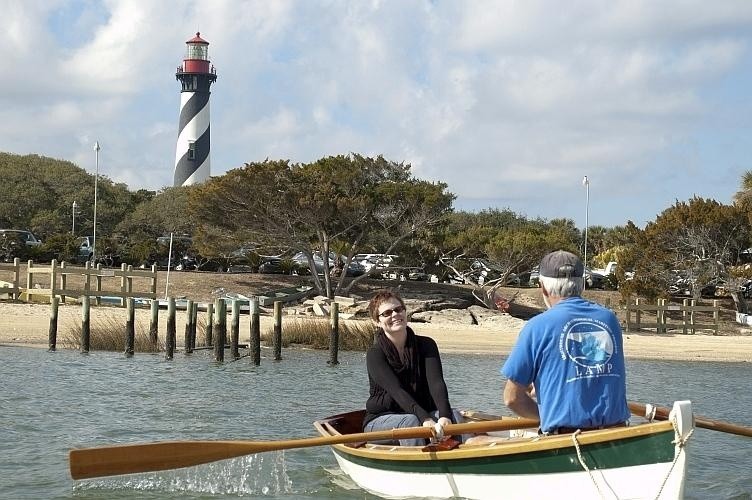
540 249 585 278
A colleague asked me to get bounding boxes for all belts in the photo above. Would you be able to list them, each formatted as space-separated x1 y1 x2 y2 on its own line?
550 421 626 433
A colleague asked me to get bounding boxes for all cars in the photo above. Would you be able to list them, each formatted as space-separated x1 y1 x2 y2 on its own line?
433 258 633 289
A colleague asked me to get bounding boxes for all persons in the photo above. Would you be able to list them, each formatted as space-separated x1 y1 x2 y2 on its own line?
361 292 473 446
498 249 630 435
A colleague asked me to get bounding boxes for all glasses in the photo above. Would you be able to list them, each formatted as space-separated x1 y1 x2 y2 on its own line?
377 306 406 317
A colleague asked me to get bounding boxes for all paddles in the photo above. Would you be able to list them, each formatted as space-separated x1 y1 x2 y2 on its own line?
70 417 542 480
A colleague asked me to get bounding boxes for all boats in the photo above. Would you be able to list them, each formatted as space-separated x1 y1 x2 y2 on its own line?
312 399 696 500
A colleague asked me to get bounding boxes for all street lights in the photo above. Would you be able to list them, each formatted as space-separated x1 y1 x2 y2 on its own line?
92 140 100 268
71 200 77 236
582 173 590 292
